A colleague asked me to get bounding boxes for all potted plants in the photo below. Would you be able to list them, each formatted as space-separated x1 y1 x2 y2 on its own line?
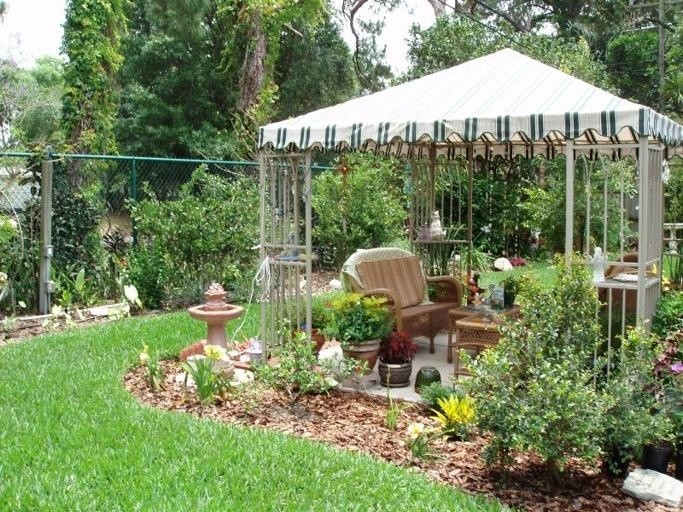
287 300 329 361
594 409 683 474
337 304 396 374
378 333 412 387
487 268 527 308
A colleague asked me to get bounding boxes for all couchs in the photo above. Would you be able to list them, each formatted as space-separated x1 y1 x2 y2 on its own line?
339 291 342 296
340 246 467 355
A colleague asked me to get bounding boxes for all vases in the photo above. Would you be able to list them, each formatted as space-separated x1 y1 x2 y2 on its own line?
412 364 443 398
